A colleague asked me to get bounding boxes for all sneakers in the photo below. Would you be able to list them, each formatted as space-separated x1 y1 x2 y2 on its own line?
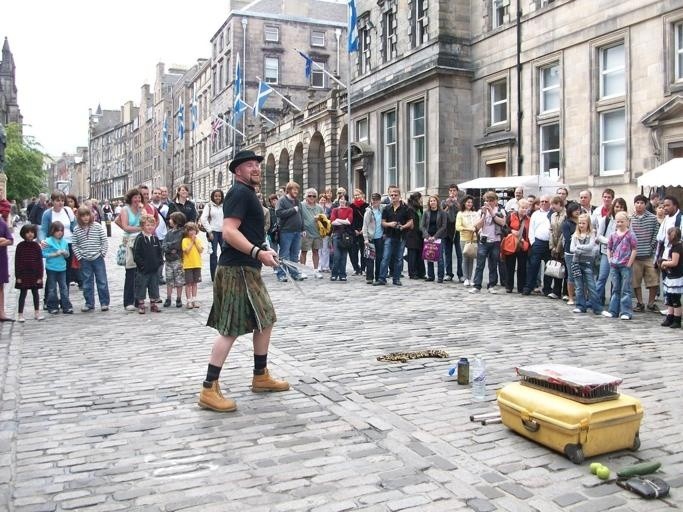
125 298 200 314
101 304 108 311
37 317 45 321
19 319 25 322
44 303 73 314
564 294 630 320
460 277 496 294
0 318 15 323
507 288 560 300
633 302 681 328
273 268 348 281
81 307 91 312
410 274 453 282
351 271 404 285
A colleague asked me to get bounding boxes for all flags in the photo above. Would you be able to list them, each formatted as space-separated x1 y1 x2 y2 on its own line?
230 51 244 115
158 95 198 153
251 75 275 123
208 110 225 144
345 1 362 55
295 48 314 79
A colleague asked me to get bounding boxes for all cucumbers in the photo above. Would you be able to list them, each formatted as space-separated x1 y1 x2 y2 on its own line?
616 461 661 478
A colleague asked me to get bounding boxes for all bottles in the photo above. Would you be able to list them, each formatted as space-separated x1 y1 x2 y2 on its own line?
472 354 486 397
456 358 470 385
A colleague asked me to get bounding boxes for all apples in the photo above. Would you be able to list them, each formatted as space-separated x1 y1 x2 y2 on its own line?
597 466 610 479
590 463 600 473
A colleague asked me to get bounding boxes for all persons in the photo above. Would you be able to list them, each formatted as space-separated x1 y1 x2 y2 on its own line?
0 182 683 324
190 148 290 413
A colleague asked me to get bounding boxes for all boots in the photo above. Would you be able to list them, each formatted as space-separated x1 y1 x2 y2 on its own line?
198 381 238 412
251 368 289 392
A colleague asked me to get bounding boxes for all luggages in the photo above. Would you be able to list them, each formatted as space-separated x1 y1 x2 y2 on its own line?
497 383 644 465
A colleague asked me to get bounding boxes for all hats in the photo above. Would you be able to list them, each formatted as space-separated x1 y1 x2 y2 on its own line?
373 193 381 200
229 151 264 173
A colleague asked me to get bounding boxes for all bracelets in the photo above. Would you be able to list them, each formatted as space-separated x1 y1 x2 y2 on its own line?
260 241 267 251
250 246 260 261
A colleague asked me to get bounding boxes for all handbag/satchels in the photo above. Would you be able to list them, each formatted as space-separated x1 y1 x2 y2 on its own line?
115 214 124 227
627 475 670 498
271 224 278 243
544 260 567 279
364 244 376 259
117 245 127 266
500 234 518 255
339 230 354 249
196 217 210 231
462 243 479 258
70 221 77 233
421 238 442 261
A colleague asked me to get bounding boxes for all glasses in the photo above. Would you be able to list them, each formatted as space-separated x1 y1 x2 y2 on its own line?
308 195 316 198
541 201 548 203
